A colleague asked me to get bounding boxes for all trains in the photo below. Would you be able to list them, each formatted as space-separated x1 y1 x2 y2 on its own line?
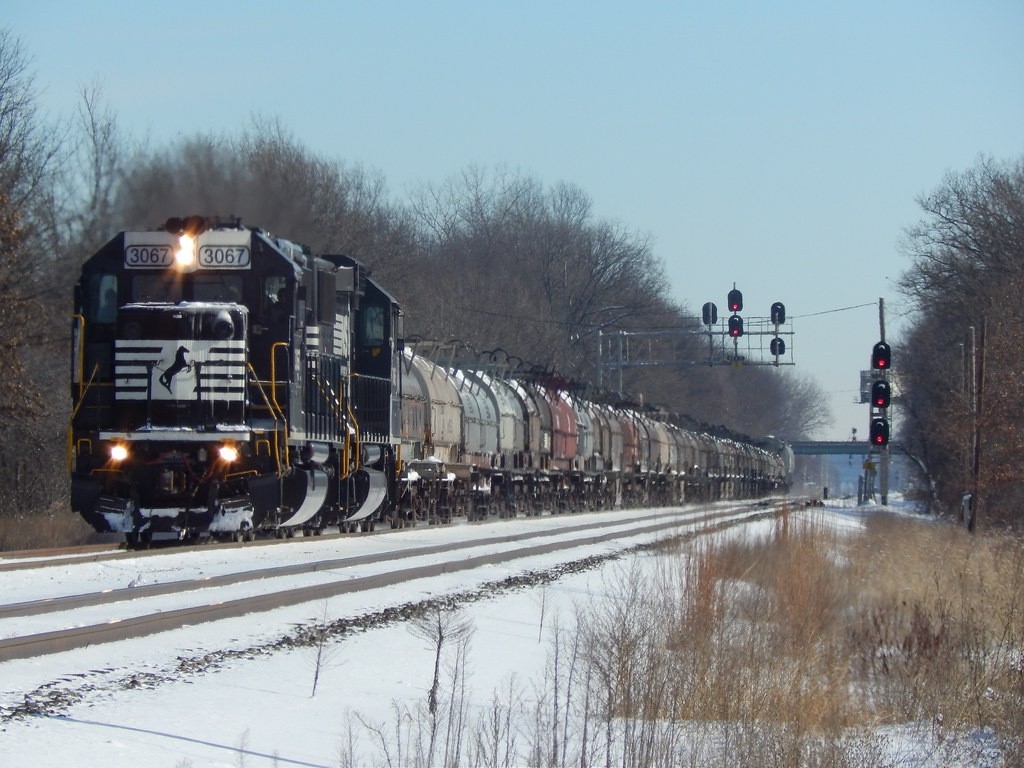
68 213 796 551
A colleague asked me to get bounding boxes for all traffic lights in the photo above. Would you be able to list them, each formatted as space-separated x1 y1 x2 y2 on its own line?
870 418 889 445
727 289 743 312
872 341 891 369
871 381 890 409
728 315 744 337
